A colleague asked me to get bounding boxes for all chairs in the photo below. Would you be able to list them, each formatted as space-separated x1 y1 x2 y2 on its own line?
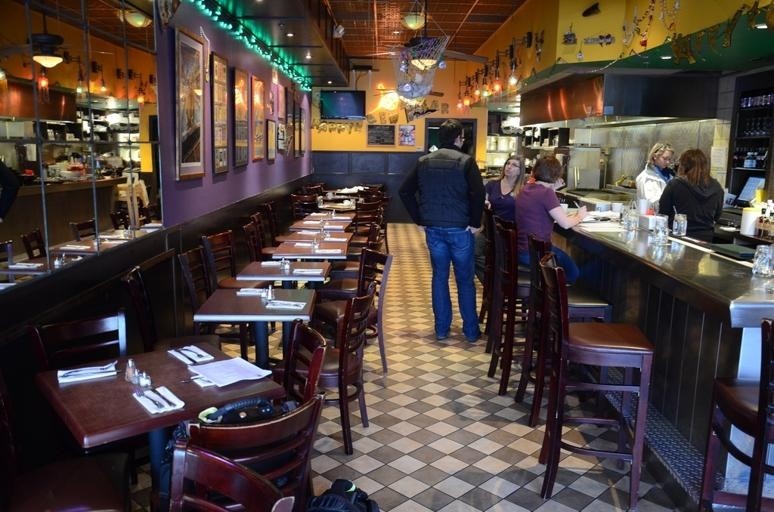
316 248 393 373
25 307 149 488
243 221 267 262
698 320 774 512
137 206 152 224
249 212 278 254
488 223 569 395
178 244 247 360
0 375 131 511
190 394 327 512
70 218 97 241
319 242 382 299
203 230 276 333
537 251 656 511
0 240 15 282
283 319 327 404
169 441 295 512
110 210 129 230
485 214 524 354
289 182 328 222
513 233 614 427
347 185 392 262
265 201 290 246
121 265 220 351
21 228 46 258
477 206 496 335
288 281 376 455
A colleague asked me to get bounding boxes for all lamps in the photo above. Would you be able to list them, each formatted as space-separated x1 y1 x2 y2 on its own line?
30 54 65 68
456 80 464 108
481 63 492 96
410 58 438 70
22 62 48 87
506 37 519 85
65 52 85 94
128 70 146 104
400 12 432 31
91 61 109 92
492 49 502 92
118 8 153 28
473 70 482 96
464 78 473 106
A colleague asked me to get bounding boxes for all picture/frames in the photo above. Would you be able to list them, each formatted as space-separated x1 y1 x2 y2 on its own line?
173 25 207 181
284 88 294 125
252 73 265 161
398 125 415 146
367 124 395 145
278 122 287 151
301 109 306 157
209 52 229 176
277 85 287 119
231 67 249 168
266 119 276 161
293 101 300 157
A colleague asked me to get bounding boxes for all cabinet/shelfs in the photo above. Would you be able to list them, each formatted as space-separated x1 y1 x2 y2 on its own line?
516 127 603 190
726 66 773 209
0 108 139 176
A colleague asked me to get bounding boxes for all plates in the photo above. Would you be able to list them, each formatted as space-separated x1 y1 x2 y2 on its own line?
187 356 272 387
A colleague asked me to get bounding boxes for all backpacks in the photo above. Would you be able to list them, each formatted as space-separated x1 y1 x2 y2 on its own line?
305 479 378 512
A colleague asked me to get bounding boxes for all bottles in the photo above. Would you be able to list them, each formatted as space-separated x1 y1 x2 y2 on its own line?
215 150 229 169
123 230 133 239
260 210 337 299
733 142 768 168
123 358 152 387
742 116 772 136
740 95 773 107
52 251 67 265
752 245 774 277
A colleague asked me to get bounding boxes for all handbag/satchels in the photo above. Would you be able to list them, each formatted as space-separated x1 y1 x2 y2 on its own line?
156 398 300 512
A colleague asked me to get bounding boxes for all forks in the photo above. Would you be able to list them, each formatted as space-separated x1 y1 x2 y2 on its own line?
63 360 118 375
181 346 207 359
134 387 164 409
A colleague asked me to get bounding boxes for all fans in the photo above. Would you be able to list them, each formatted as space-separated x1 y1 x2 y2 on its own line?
367 0 489 64
1 2 113 56
371 59 444 96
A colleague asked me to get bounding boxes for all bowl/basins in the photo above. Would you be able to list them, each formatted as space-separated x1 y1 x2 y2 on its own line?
60 171 80 177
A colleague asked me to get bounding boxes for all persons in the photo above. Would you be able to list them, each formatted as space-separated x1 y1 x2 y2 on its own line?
513 155 588 283
657 147 724 243
397 118 486 343
634 140 677 213
472 153 525 299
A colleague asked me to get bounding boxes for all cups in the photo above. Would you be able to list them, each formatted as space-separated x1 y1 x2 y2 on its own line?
560 203 640 230
654 214 668 245
673 214 687 236
318 193 364 210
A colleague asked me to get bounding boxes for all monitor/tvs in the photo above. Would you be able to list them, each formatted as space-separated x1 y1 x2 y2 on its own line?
320 89 366 120
732 175 766 205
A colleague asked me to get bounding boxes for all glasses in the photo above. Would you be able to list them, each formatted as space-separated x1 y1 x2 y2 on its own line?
506 161 519 167
461 136 467 142
